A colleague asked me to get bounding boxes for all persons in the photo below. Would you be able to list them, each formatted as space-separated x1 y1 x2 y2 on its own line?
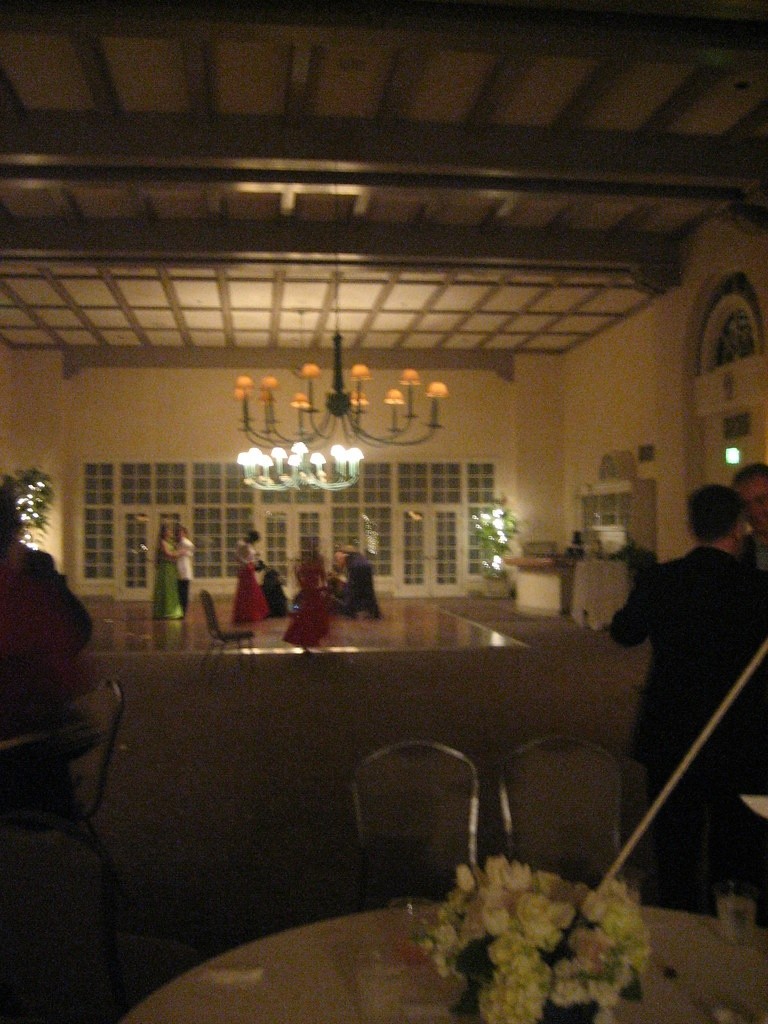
0 486 95 820
608 462 768 925
151 525 196 623
232 529 385 656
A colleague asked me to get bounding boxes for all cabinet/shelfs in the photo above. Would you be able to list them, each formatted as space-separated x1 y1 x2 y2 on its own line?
576 474 659 559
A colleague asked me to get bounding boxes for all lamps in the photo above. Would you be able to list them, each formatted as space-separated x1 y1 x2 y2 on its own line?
234 304 365 497
231 149 452 454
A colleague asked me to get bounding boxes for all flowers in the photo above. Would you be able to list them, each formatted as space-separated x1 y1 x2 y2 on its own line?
413 855 656 1024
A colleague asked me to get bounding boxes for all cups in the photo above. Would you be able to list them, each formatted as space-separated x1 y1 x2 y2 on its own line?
386 896 443 972
712 878 759 944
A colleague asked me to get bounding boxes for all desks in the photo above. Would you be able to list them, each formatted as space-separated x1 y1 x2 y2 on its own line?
111 900 767 1022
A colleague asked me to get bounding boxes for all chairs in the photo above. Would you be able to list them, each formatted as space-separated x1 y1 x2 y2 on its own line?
492 736 628 896
196 588 261 687
345 740 480 919
0 675 132 911
